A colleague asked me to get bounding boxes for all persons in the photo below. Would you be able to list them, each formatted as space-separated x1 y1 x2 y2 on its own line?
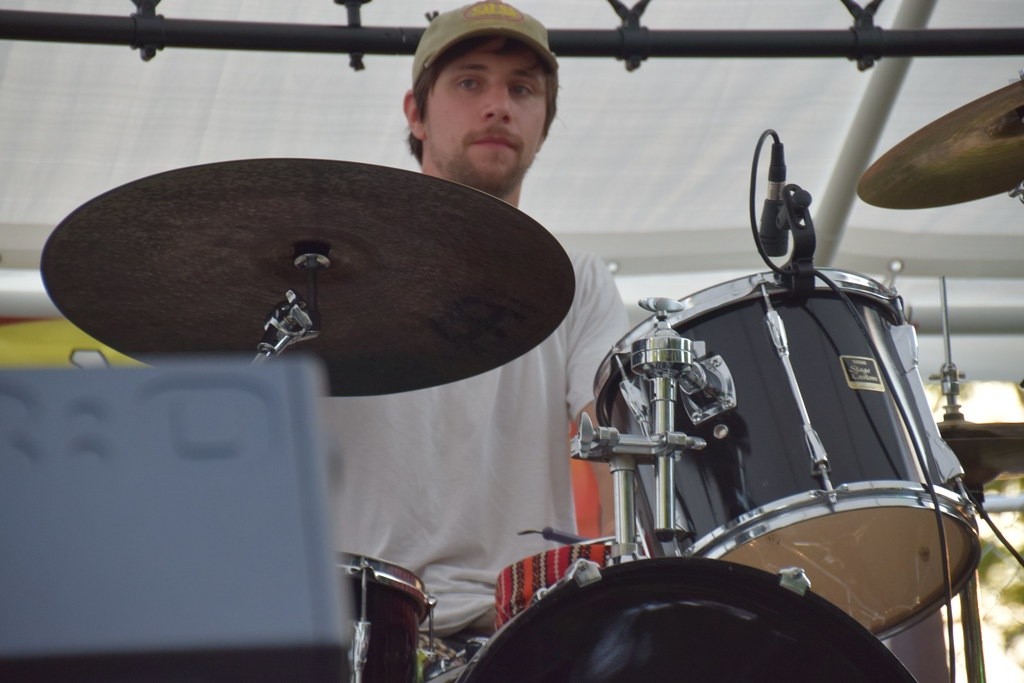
321 0 633 637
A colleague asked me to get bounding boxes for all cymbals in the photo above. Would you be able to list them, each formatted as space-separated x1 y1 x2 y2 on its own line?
448 557 920 683
39 157 575 397
856 80 1024 211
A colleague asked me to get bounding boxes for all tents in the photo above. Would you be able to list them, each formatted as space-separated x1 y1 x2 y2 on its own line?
2 0 1024 380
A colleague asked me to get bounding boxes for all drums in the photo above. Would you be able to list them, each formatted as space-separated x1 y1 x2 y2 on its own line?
337 550 431 683
592 268 981 640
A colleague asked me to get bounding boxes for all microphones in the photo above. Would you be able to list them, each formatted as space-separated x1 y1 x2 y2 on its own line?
759 143 789 256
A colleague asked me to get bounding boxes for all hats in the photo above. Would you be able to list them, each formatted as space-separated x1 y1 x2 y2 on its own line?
412 0 559 89
